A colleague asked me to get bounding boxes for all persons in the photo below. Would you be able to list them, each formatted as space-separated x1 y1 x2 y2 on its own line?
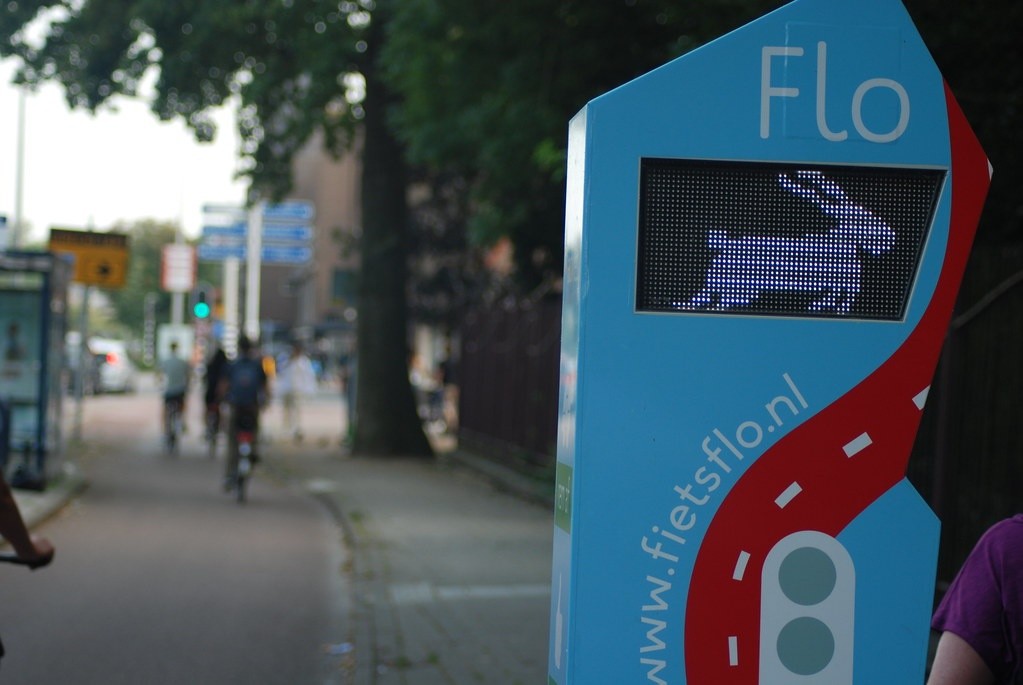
924 513 1022 685
156 331 321 490
1 465 54 568
